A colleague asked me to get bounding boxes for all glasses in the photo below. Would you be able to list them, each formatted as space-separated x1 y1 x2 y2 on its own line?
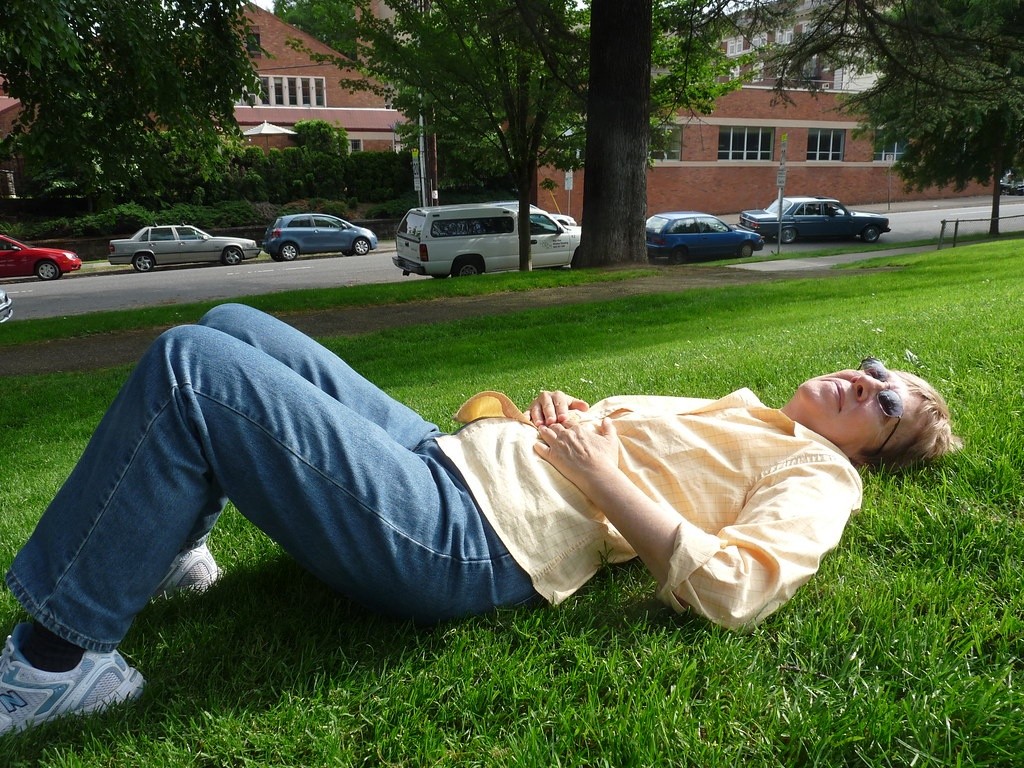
856 356 904 457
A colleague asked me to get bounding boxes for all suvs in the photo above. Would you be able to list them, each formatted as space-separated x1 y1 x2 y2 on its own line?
391 207 582 279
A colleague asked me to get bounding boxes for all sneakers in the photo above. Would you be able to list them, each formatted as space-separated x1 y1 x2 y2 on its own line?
0 620 145 740
139 542 225 615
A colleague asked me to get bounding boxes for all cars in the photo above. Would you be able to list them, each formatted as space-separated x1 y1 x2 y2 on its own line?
645 210 764 265
107 221 261 273
262 213 379 262
486 200 578 227
0 285 13 326
998 173 1024 196
736 195 891 245
0 234 82 281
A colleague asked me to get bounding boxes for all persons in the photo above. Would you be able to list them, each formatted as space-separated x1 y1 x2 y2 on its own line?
0 300 959 743
